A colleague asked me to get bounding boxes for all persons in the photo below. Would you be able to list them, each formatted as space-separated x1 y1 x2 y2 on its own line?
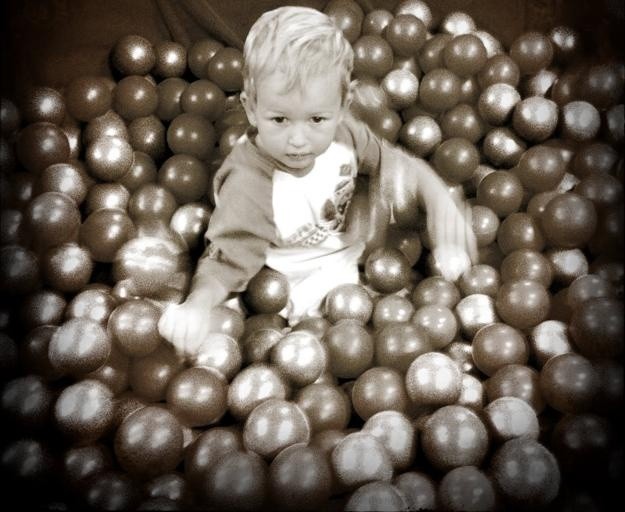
158 6 469 354
